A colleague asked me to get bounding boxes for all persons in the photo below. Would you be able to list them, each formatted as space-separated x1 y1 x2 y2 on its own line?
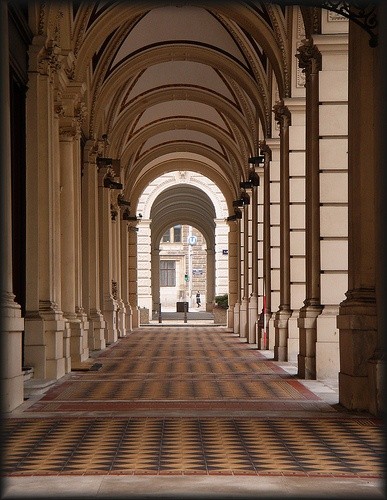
196 291 202 308
